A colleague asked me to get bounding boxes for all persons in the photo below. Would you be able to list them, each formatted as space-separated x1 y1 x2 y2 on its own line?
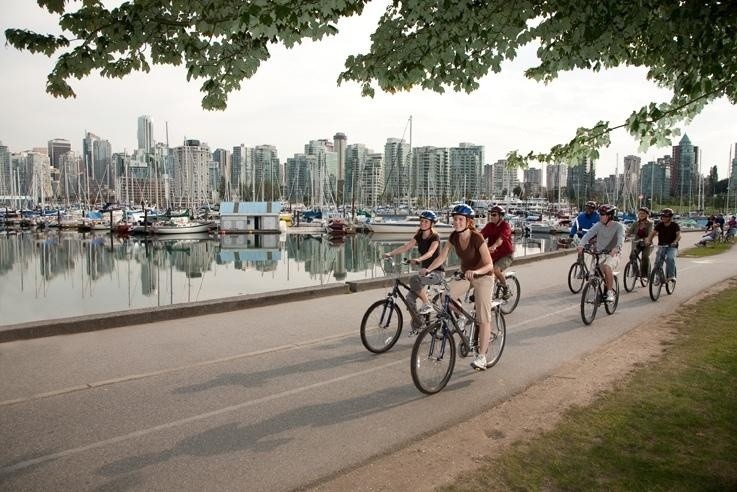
694 212 736 249
625 207 654 283
480 206 514 300
381 210 444 338
418 205 493 372
565 201 599 237
644 208 681 283
576 204 625 302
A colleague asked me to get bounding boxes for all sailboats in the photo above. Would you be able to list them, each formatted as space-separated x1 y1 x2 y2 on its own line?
220 115 736 233
366 232 456 277
0 136 219 234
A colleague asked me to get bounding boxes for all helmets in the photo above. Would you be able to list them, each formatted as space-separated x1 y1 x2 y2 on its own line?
419 211 438 222
489 206 506 213
450 204 476 219
585 201 598 209
656 208 674 218
638 206 651 216
597 204 617 215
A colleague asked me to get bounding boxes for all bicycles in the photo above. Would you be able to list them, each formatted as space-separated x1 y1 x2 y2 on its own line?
449 265 521 315
411 268 506 395
566 239 601 295
578 244 620 329
359 252 459 355
644 234 679 302
620 234 654 295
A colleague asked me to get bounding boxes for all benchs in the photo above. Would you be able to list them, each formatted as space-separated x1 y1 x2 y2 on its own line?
705 231 721 247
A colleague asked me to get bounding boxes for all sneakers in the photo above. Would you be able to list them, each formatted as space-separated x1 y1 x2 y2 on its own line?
408 328 418 337
606 290 615 301
470 354 487 371
454 315 469 331
502 284 513 299
418 302 433 315
